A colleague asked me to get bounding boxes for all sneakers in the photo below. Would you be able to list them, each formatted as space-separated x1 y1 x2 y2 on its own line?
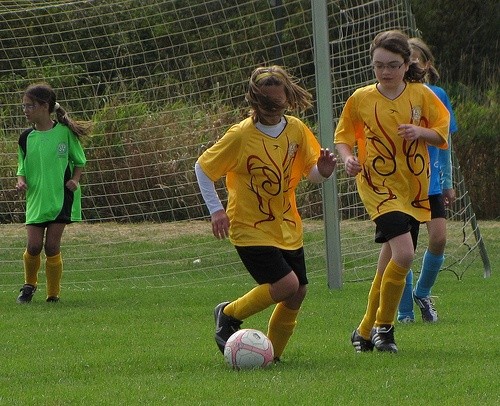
214 301 243 357
398 316 414 323
412 292 439 322
47 296 58 303
351 330 375 354
370 323 397 354
16 283 36 303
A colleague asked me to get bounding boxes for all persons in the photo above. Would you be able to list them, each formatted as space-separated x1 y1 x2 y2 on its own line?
16 84 93 302
334 30 450 353
397 38 458 323
195 66 336 362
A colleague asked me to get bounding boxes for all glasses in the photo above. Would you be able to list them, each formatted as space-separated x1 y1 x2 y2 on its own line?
370 61 405 72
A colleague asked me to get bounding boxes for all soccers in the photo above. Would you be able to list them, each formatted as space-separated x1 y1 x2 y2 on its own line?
224 329 274 369
